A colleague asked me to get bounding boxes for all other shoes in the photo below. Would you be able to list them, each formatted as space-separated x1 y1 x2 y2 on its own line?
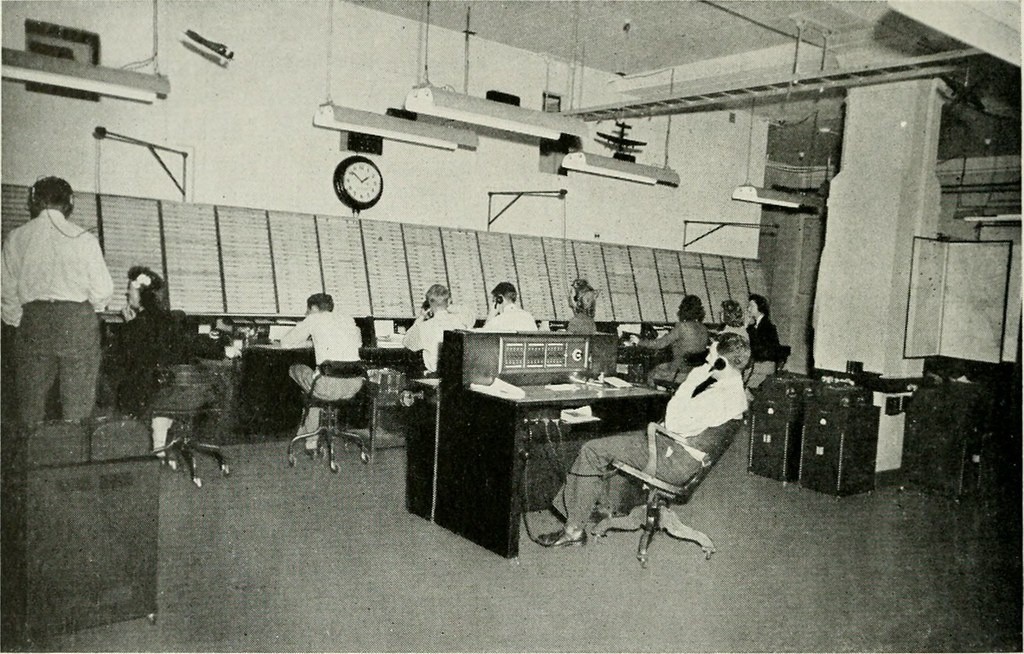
303 446 321 461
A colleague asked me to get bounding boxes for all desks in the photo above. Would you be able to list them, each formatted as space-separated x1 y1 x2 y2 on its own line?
404 377 673 559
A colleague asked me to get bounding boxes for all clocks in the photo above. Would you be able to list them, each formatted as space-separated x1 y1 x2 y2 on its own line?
332 155 383 210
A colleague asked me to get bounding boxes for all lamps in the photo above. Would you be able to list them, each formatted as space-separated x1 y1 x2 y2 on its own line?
1 0 1022 225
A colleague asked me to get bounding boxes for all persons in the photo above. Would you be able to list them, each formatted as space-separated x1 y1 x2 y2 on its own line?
481 281 539 332
629 294 780 394
279 292 364 461
535 332 752 549
1 176 116 424
102 266 187 418
401 283 476 406
567 278 597 332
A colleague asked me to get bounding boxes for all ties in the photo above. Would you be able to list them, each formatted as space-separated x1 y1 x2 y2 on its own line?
690 376 718 398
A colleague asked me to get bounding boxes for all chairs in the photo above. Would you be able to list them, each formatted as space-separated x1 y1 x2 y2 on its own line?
651 345 793 395
287 359 381 473
589 374 786 569
149 360 231 488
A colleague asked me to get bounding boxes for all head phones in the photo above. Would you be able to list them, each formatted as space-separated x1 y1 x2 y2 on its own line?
29 180 74 216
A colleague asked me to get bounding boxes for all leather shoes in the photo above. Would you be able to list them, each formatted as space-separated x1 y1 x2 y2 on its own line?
534 526 588 547
